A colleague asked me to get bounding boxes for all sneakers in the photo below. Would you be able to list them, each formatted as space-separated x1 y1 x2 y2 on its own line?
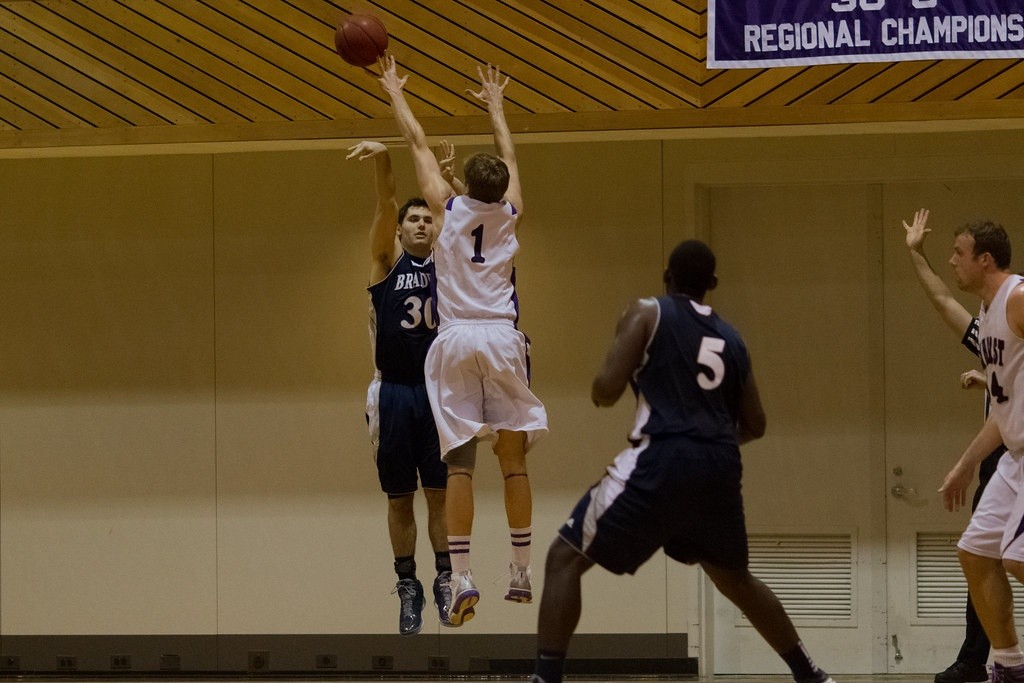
391 578 426 637
504 561 532 602
439 569 480 626
431 571 455 627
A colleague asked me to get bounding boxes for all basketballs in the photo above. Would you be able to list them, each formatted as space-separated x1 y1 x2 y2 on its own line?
334 13 388 67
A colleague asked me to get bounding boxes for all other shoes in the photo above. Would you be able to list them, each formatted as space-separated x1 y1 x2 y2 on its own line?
934 661 989 683
795 668 836 683
991 662 1024 683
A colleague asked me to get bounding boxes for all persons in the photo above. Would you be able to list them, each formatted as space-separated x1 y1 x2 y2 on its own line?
902 208 1024 683
345 49 549 637
529 238 835 683
